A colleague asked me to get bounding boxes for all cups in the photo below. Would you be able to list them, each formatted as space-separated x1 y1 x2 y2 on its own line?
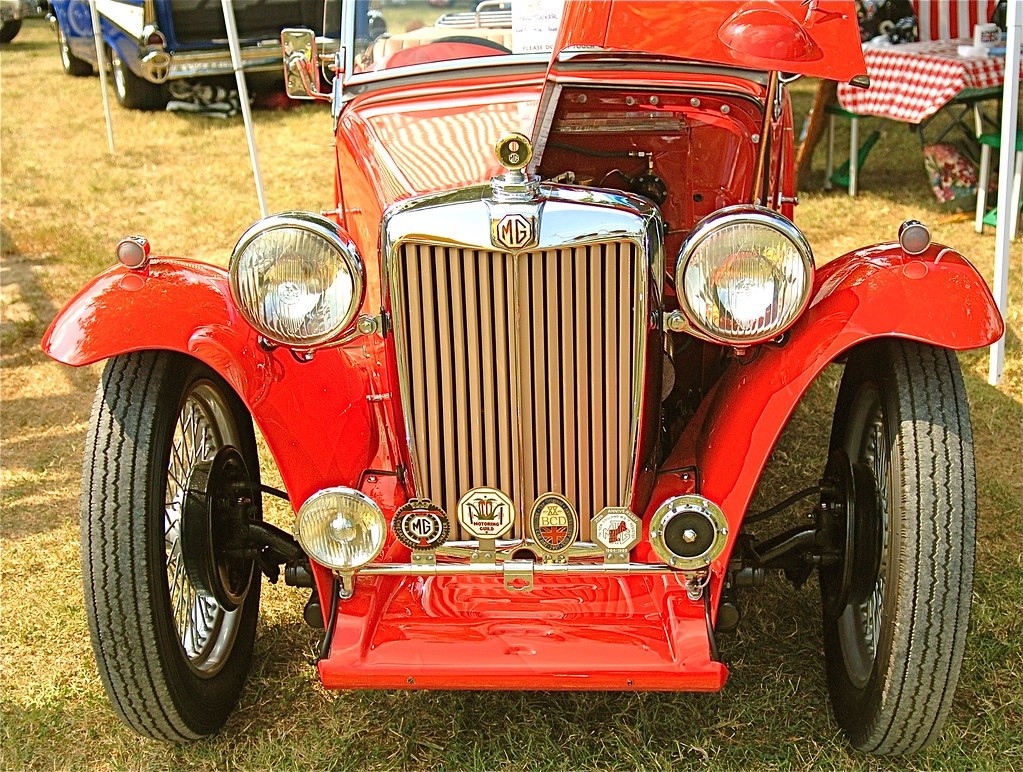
973 23 1002 49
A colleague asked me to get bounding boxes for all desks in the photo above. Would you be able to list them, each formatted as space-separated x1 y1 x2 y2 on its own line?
838 37 1023 197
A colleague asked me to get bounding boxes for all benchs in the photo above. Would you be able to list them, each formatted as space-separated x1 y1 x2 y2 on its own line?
372 33 512 63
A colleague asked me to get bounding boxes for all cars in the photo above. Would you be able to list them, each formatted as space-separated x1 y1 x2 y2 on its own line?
37 0 1006 763
0 0 388 112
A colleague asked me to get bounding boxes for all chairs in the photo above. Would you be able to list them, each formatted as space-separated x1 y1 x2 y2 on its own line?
910 0 1023 239
